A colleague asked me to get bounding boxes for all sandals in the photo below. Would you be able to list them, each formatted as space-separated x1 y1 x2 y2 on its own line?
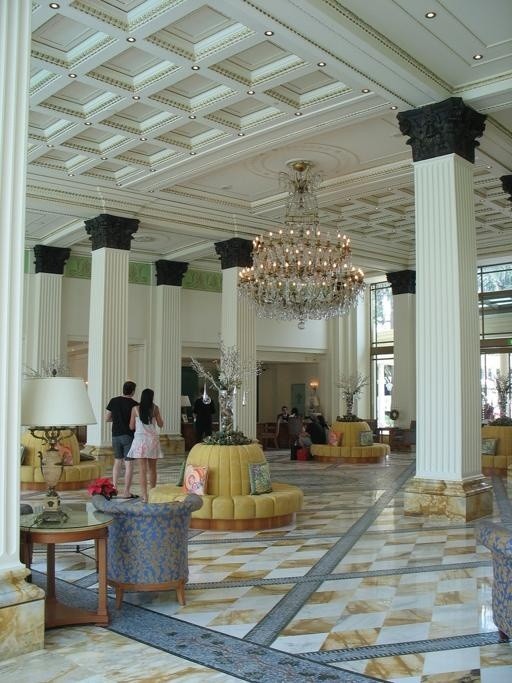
122 493 139 499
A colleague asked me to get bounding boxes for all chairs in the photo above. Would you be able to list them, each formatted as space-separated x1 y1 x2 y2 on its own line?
288 417 304 447
90 494 202 608
261 417 281 448
473 474 512 641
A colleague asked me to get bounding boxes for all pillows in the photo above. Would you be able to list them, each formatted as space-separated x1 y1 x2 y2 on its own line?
481 437 500 455
247 462 273 493
360 432 374 445
20 445 27 465
328 431 342 445
52 443 74 466
184 465 209 497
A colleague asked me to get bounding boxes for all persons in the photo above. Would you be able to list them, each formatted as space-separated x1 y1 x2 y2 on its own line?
277 406 329 444
193 386 216 443
104 382 163 502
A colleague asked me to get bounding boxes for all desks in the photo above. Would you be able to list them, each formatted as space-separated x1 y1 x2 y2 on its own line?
21 510 110 628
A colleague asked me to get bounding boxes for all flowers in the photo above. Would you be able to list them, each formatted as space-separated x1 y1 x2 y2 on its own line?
88 476 119 501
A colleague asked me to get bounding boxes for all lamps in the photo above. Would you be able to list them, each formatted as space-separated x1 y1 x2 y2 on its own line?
20 378 97 522
310 381 319 393
180 396 191 423
237 161 365 329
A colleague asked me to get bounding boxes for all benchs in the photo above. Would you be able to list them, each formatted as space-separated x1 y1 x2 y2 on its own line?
308 443 392 464
147 483 305 532
482 454 512 476
19 461 105 493
